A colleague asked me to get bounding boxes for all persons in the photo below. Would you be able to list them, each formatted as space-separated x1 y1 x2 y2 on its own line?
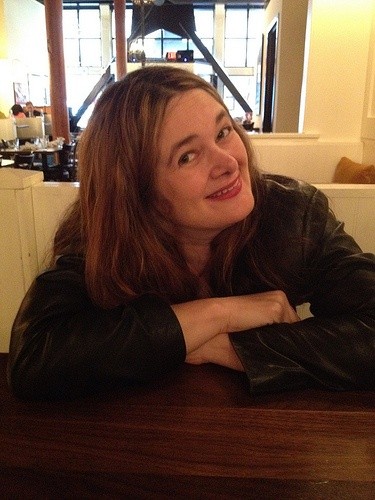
6 66 375 403
11 101 42 119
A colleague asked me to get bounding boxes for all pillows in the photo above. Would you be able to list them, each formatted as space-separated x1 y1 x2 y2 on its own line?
334 157 375 184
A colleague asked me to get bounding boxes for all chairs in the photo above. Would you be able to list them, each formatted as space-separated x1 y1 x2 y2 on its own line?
0 106 51 144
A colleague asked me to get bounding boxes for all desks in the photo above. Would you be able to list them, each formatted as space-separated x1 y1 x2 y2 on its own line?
0 361 375 500
0 145 63 168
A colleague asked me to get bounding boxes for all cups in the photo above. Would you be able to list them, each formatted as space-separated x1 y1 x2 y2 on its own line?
13 138 19 150
57 136 63 148
34 135 49 150
